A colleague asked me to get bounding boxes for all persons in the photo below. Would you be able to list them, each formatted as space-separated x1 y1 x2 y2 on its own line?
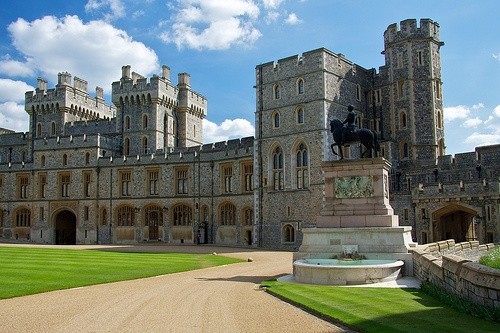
196 229 201 244
338 104 358 147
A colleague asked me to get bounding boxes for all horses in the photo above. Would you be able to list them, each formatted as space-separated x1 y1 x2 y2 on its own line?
329 119 382 160
